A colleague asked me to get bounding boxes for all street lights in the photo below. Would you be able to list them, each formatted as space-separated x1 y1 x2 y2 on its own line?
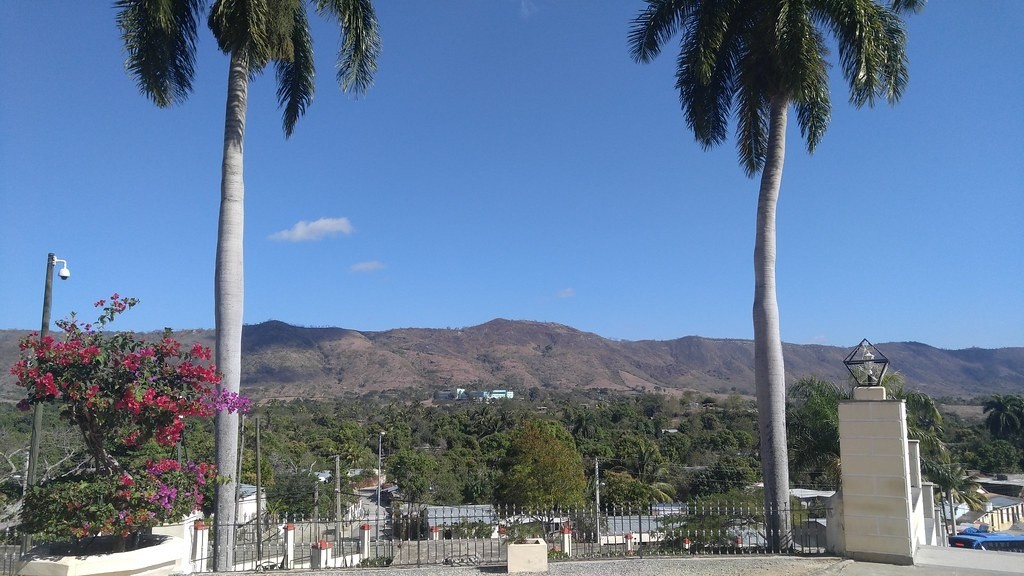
375 431 385 562
19 254 72 558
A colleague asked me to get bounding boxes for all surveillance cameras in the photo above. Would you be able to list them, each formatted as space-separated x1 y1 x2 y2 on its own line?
58 269 70 280
